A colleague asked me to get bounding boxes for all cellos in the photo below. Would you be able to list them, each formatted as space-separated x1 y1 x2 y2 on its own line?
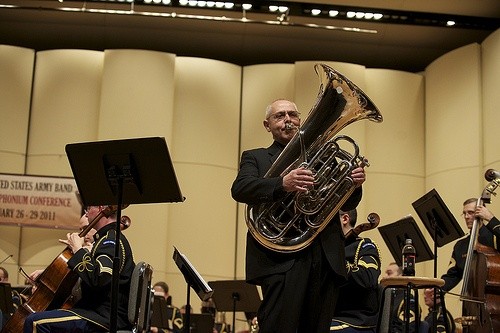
1 204 131 333
458 168 500 332
344 214 381 245
89 215 132 240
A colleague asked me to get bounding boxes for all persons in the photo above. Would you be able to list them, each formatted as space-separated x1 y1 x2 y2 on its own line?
147 282 183 333
327 207 382 333
229 98 366 333
428 198 500 302
0 267 20 333
378 261 465 333
22 205 136 333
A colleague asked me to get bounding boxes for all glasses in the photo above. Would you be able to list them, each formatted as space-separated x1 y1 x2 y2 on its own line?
266 112 302 121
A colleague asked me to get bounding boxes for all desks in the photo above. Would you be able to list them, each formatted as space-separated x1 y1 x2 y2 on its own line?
375 277 450 333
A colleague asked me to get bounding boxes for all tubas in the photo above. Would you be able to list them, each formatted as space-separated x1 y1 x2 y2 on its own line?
243 64 384 254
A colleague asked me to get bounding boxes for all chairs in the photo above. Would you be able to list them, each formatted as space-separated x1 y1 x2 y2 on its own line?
107 261 153 333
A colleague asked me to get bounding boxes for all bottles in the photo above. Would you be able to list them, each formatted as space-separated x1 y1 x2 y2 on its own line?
403 239 416 276
